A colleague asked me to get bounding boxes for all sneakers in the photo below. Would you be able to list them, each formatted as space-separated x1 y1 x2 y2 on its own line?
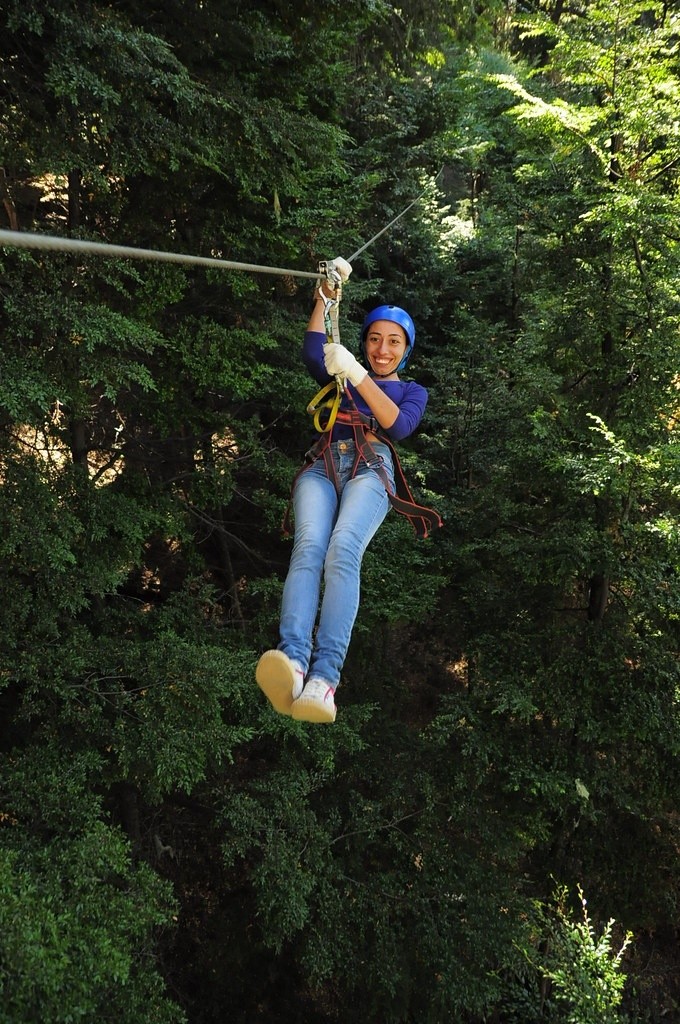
291 677 340 723
256 646 307 719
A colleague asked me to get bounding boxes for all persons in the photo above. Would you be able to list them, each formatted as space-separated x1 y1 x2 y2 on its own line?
256 257 428 722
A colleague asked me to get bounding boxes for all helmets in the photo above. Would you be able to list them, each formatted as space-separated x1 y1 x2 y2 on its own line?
365 304 415 348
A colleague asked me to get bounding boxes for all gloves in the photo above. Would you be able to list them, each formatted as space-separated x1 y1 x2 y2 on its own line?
323 342 369 387
313 257 352 299
